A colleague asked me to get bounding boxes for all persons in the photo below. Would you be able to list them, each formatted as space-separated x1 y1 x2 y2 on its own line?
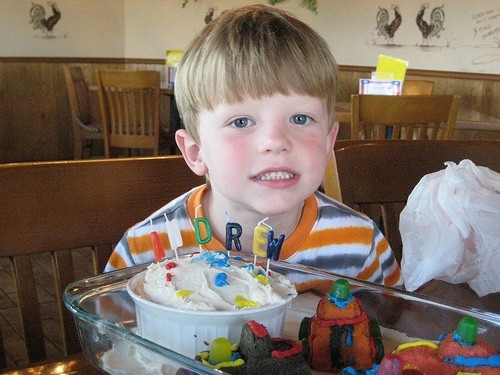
93 3 405 342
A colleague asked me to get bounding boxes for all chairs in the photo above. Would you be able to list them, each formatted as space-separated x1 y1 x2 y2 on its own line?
95 68 161 159
0 156 205 370
63 64 104 159
323 139 500 269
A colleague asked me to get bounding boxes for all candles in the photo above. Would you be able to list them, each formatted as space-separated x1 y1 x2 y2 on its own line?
148 203 285 262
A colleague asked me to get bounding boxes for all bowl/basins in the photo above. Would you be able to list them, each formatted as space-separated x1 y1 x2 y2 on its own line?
125 270 298 368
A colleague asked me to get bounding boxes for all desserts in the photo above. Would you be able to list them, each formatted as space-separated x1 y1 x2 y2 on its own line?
125 251 299 368
174 279 500 375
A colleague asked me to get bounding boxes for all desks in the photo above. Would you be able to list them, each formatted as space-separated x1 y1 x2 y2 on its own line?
86 81 179 155
351 93 463 140
332 100 500 133
